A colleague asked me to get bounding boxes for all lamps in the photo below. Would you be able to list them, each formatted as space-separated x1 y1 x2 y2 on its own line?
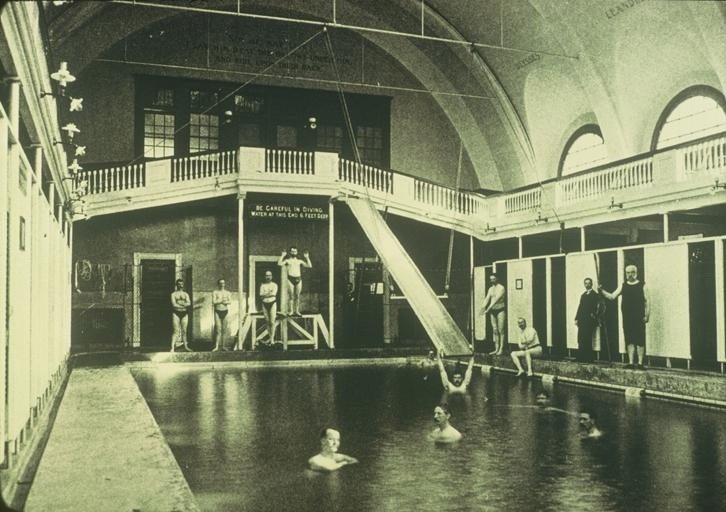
50 61 88 221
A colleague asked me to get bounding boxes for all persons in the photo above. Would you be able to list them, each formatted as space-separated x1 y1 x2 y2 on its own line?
434 343 476 396
275 246 312 317
596 263 650 371
573 278 602 363
210 278 230 353
340 282 357 332
508 316 544 378
478 272 505 357
428 401 461 442
496 389 580 417
306 425 361 473
168 279 195 352
416 350 440 367
574 409 601 439
257 269 278 347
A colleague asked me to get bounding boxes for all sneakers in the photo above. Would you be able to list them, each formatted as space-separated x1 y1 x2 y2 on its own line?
622 364 647 371
